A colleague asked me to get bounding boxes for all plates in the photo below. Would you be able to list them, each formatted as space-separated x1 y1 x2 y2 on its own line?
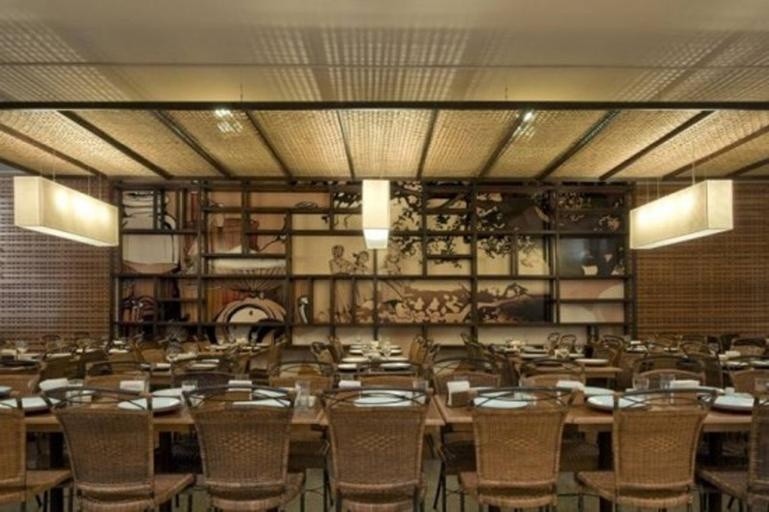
0 342 767 413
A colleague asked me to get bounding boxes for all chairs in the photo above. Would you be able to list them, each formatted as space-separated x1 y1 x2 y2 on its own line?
1 330 769 512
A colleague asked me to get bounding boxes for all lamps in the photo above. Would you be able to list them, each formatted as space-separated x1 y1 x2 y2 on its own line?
12 135 123 250
360 177 392 251
627 144 735 252
